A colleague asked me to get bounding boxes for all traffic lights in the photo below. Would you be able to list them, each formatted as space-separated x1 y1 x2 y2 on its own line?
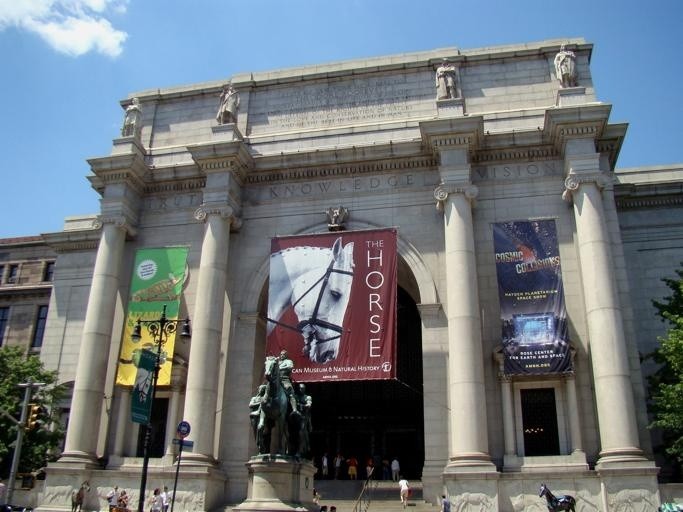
25 404 42 431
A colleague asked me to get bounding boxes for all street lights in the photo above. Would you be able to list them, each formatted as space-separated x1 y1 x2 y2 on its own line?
132 304 190 512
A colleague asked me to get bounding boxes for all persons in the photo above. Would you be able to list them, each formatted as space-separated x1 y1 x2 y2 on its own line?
554 44 577 87
151 488 164 512
122 98 143 138
399 477 410 507
219 87 240 124
160 487 172 512
249 385 269 453
278 350 300 417
321 452 400 481
441 495 450 512
118 490 128 507
320 506 327 512
296 384 312 456
107 486 120 512
330 505 336 511
313 488 319 505
435 57 457 99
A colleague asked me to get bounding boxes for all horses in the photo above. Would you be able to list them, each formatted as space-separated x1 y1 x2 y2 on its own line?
69 481 91 512
538 481 576 512
266 235 356 365
254 355 291 453
